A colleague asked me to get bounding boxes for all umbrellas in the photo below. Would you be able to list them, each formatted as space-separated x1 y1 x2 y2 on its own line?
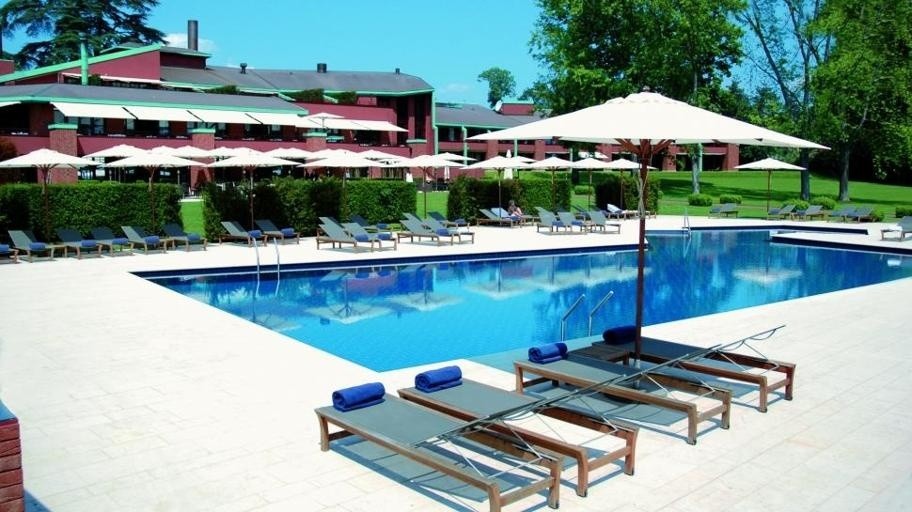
302 112 345 133
462 85 832 362
734 157 807 214
0 147 104 242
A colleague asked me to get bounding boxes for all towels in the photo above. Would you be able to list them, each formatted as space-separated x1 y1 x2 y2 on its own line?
415 365 463 393
603 326 637 345
332 383 385 412
528 343 568 364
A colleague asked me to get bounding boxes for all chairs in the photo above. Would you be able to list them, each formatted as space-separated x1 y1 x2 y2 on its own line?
881 216 912 241
592 324 797 413
767 206 876 224
513 344 733 445
709 202 738 219
397 374 640 497
314 391 564 512
